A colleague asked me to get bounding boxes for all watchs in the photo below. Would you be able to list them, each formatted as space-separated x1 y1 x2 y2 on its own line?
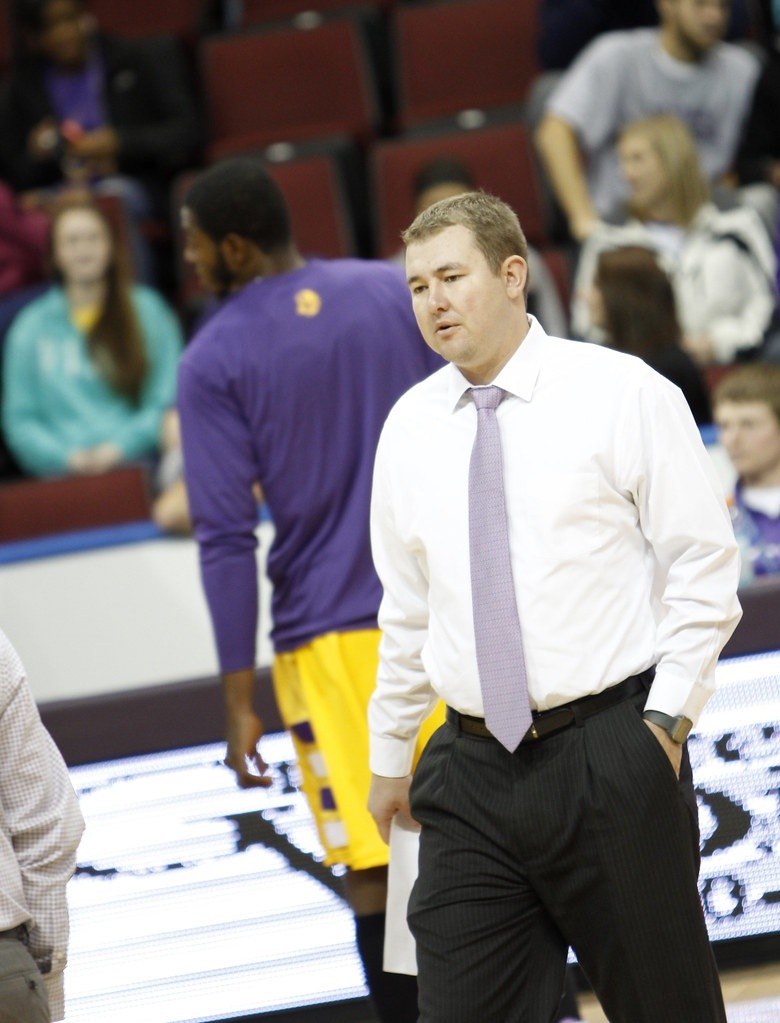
642 710 693 743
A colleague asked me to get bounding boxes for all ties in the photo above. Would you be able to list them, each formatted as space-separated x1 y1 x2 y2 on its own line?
468 386 533 753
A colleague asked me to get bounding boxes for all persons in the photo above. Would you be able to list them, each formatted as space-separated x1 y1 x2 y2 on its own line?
366 192 741 1023
179 159 450 1023
0 0 780 594
0 627 86 1023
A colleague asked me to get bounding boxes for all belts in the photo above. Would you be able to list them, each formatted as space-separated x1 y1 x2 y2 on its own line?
445 668 655 742
0 925 28 939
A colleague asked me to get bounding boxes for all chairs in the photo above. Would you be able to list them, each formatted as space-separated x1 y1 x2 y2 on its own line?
0 0 598 543
